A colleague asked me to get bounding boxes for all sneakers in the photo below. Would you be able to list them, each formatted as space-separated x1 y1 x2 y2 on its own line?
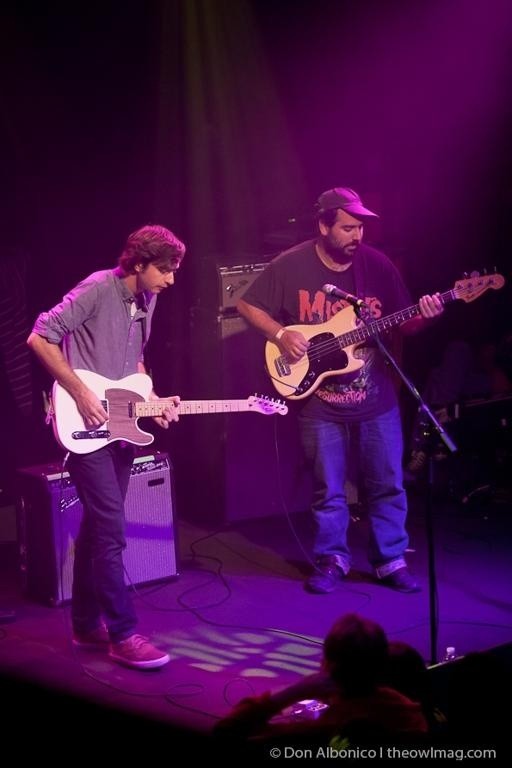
372 564 423 595
107 632 172 671
305 551 348 595
71 622 127 650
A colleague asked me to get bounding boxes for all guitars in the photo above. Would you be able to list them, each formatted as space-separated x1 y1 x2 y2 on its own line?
50 369 289 455
265 268 505 399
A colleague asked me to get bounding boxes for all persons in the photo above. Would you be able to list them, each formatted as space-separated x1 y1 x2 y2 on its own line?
236 185 448 595
27 224 184 670
210 608 512 768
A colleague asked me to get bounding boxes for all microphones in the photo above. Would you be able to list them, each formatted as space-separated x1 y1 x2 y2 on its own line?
319 280 365 310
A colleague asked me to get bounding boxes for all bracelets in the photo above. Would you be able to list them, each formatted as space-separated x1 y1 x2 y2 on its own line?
274 328 286 345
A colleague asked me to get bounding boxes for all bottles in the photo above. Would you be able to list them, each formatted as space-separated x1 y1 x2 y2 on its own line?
443 647 458 662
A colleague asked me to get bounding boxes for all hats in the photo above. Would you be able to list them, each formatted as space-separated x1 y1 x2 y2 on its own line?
316 186 381 222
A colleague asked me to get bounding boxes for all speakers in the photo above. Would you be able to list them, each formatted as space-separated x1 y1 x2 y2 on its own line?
12 453 181 608
188 313 359 527
